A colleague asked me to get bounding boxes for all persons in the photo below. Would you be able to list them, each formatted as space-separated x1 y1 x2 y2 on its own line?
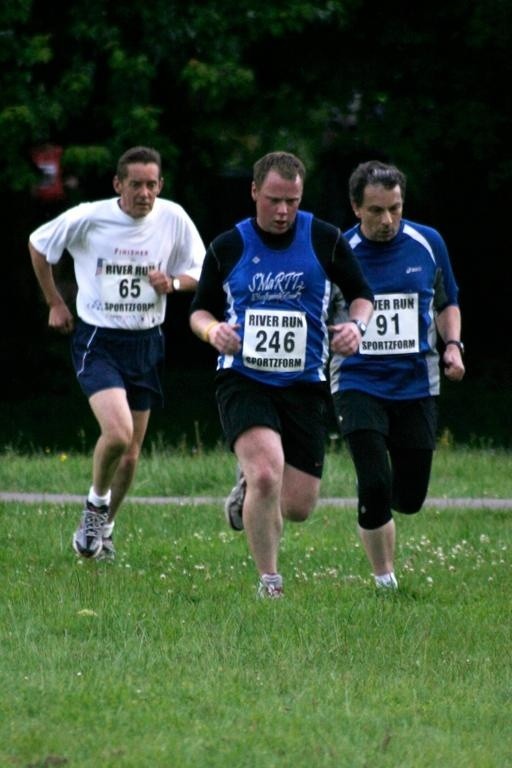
328 157 467 593
29 145 208 559
187 149 376 601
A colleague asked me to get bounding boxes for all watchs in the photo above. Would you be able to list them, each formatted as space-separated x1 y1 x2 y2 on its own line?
170 274 181 293
444 339 465 356
350 318 368 337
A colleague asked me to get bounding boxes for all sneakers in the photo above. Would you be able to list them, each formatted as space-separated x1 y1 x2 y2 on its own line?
71 499 109 559
256 572 285 600
224 461 247 532
100 536 117 560
